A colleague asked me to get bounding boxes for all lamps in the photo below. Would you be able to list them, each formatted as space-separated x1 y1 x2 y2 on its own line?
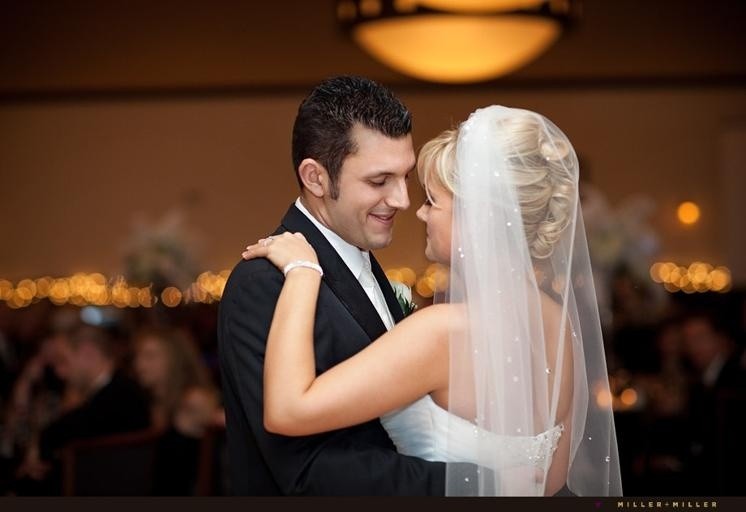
337 0 579 84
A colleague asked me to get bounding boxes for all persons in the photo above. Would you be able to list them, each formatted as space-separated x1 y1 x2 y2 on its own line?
216 72 555 499
0 257 746 492
233 104 628 495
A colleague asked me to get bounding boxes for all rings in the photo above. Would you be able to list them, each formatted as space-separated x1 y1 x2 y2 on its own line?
263 236 274 247
